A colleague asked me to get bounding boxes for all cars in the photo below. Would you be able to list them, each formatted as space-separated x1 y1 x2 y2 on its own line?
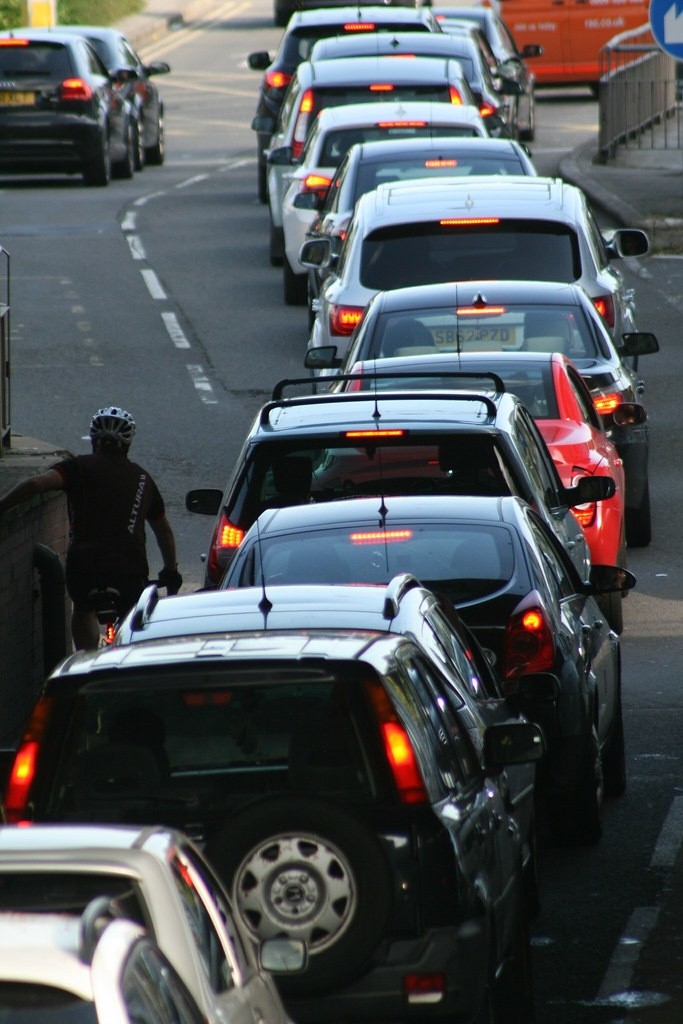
41 23 170 169
0 26 146 187
209 491 638 817
0 898 219 1024
16 629 538 1015
0 820 305 1022
247 0 661 562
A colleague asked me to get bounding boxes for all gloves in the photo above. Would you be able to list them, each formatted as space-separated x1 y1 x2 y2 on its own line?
164 562 182 596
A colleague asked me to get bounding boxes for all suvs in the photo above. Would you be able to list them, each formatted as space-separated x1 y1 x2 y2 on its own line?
186 369 617 611
116 572 544 859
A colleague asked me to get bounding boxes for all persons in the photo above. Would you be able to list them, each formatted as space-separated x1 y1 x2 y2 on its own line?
0 406 183 652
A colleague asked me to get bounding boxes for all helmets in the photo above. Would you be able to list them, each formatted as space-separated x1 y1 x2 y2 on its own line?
90 405 136 453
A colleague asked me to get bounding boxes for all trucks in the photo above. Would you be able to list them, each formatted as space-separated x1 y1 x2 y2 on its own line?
482 0 663 100
